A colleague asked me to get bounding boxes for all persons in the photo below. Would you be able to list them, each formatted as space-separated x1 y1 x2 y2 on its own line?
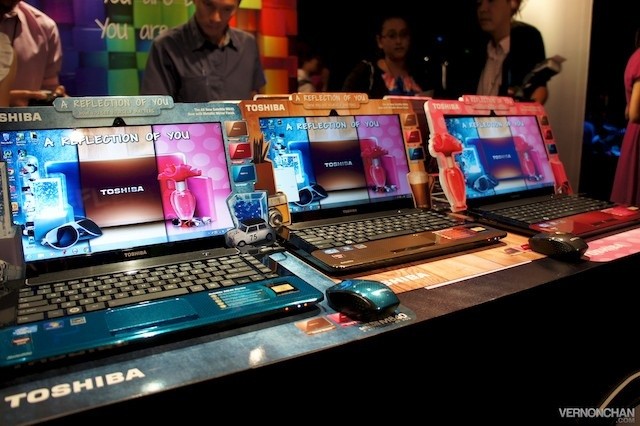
611 49 640 207
345 13 444 99
141 0 267 103
297 53 329 93
453 0 549 107
0 0 70 107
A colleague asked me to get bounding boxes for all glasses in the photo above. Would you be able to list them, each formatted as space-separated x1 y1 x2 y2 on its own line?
41 217 102 250
472 174 498 191
292 183 327 207
382 30 409 39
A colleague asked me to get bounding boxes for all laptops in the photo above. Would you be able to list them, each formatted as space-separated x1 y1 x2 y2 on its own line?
424 95 640 240
237 92 507 272
0 95 324 379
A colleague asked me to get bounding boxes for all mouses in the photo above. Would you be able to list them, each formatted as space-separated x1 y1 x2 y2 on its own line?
325 279 400 321
529 232 589 261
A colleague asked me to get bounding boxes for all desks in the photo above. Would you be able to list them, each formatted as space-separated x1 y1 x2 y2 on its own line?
0 220 640 426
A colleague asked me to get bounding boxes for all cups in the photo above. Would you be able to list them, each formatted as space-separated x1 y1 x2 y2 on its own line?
406 172 435 209
268 195 291 225
